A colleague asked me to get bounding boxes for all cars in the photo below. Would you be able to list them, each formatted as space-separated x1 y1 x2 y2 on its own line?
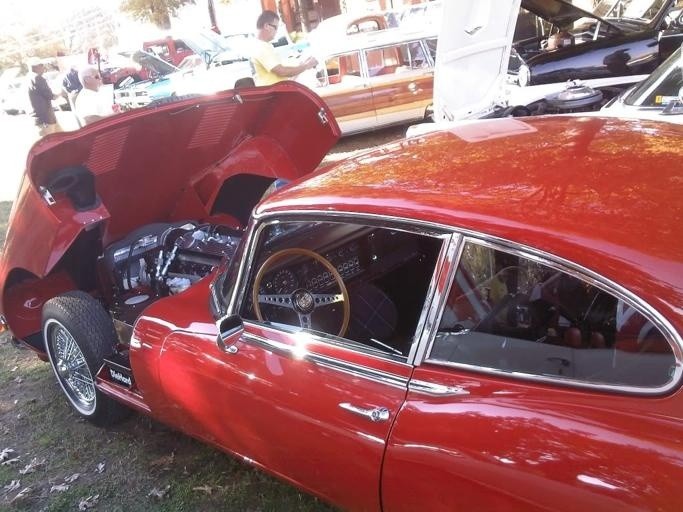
507 0 681 87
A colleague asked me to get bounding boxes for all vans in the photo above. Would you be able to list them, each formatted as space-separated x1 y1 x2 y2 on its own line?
603 43 683 124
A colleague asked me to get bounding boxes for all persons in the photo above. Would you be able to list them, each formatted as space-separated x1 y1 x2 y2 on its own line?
58 66 83 130
25 57 65 138
73 65 116 128
243 8 319 88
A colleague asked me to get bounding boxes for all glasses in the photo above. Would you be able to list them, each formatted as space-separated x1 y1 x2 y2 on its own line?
268 23 277 30
90 75 99 80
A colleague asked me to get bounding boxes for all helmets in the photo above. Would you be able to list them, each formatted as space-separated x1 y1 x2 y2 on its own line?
31 57 46 66
